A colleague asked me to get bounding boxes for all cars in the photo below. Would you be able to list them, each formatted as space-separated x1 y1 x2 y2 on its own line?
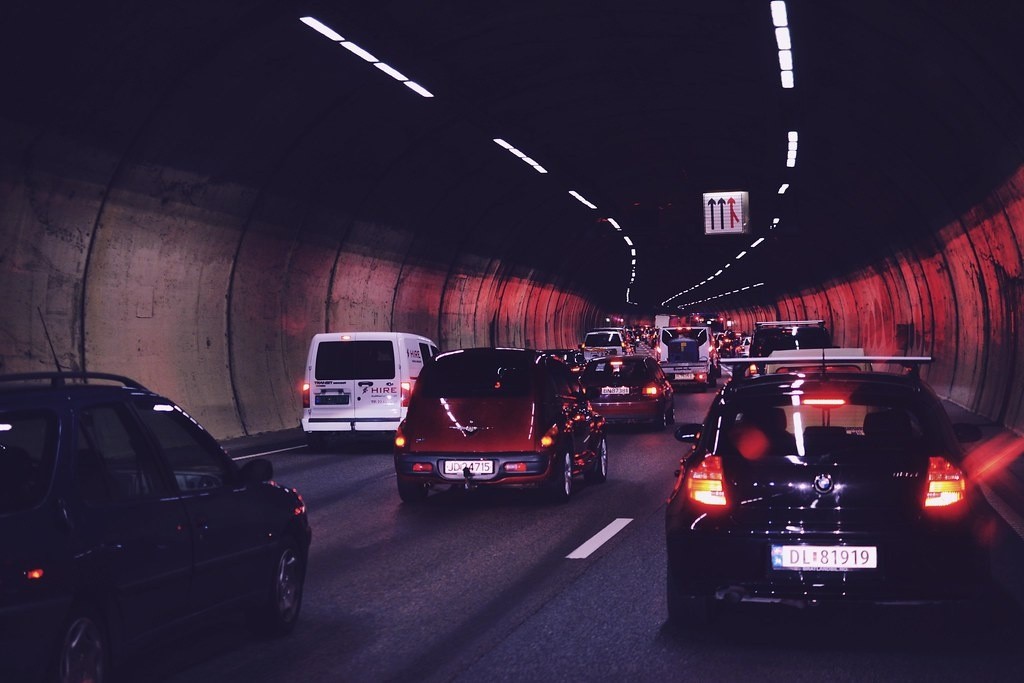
393 348 676 502
715 330 752 358
762 347 876 377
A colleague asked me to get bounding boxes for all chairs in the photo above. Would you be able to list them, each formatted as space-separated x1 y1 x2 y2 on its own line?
751 406 799 458
859 408 914 467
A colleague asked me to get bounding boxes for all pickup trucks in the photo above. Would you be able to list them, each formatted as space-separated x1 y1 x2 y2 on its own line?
655 326 722 387
579 330 627 362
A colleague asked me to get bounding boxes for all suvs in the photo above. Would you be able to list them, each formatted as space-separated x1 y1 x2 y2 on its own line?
0 370 312 682
668 356 978 609
748 319 836 358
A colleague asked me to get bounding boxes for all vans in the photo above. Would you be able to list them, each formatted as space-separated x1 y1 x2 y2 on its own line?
299 331 441 433
